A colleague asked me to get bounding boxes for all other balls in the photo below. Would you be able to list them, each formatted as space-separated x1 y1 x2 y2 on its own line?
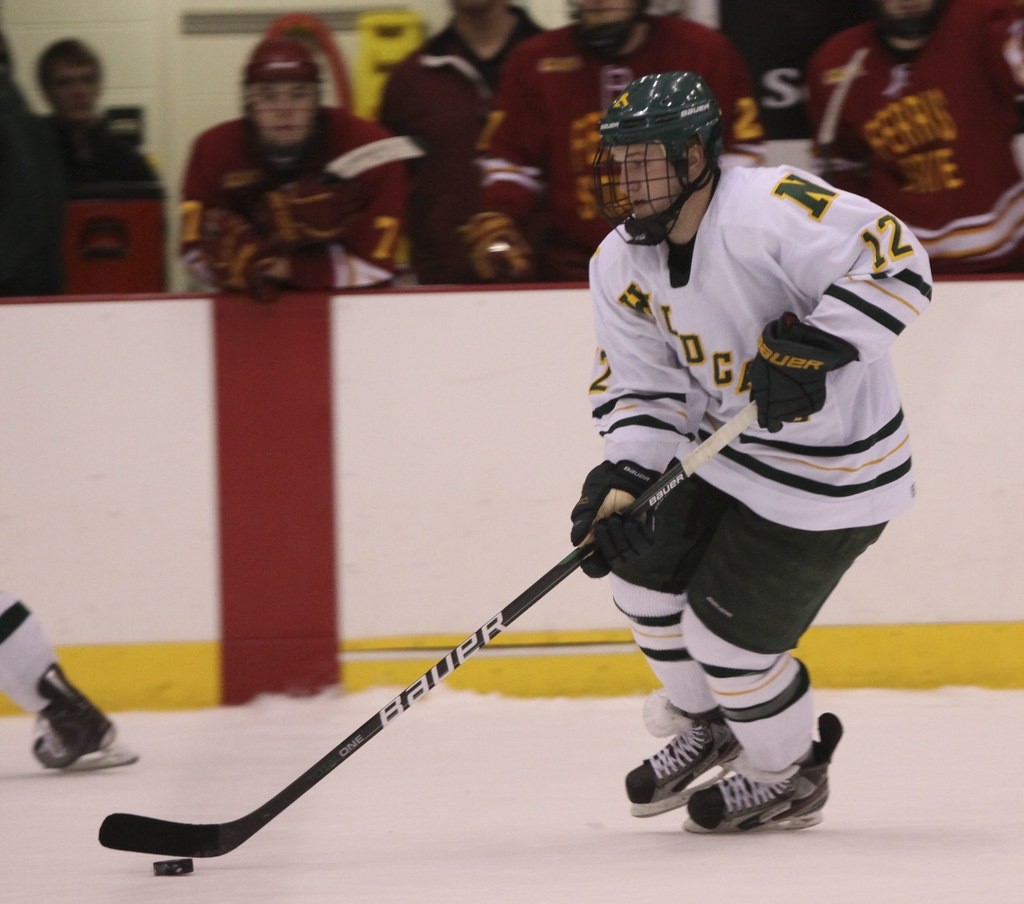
152 857 195 877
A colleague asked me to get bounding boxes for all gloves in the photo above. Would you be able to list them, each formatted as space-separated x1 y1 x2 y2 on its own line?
203 213 290 301
252 178 371 246
471 212 532 283
571 461 665 579
749 311 858 433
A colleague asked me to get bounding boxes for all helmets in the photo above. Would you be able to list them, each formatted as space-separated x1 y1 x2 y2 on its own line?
593 70 721 245
243 39 323 168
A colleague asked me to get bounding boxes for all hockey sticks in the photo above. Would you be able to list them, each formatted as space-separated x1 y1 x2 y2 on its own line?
97 401 761 858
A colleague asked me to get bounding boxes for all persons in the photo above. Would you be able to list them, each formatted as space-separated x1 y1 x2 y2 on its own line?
177 37 413 304
0 31 168 298
570 70 933 833
0 584 118 769
377 0 552 287
456 0 767 284
801 0 1024 280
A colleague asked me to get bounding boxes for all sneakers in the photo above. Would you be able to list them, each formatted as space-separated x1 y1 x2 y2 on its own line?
33 663 142 772
681 713 843 833
625 694 743 819
